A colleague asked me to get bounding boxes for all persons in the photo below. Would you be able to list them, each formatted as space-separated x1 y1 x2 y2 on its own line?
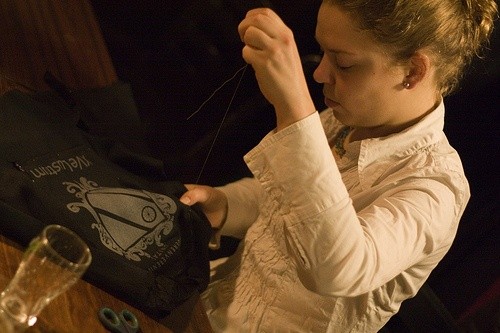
179 0 498 332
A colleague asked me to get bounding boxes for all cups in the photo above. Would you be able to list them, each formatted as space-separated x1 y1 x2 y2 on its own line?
1 224 93 327
